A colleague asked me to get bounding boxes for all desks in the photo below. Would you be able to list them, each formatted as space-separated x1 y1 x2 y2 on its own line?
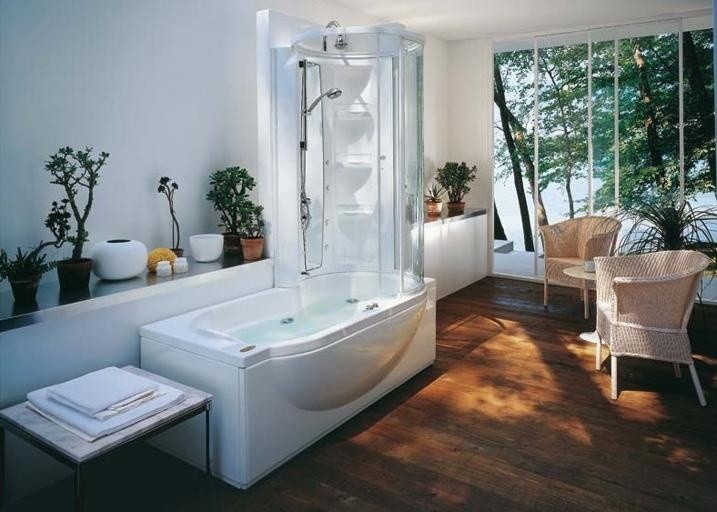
561 264 607 345
0 365 216 512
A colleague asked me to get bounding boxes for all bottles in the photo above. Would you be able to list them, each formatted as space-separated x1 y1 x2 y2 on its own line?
156 258 188 277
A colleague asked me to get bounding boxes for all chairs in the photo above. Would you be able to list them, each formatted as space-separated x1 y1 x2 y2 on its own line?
593 248 710 406
538 215 621 320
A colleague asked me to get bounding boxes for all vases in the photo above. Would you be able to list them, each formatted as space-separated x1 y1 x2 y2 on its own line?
90 239 149 282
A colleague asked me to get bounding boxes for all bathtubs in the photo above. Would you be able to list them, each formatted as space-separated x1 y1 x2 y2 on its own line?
140 271 437 492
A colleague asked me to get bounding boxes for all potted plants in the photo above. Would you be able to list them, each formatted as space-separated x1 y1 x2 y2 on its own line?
0 240 59 303
237 200 265 260
424 182 447 216
42 144 110 292
157 175 183 257
207 163 256 256
435 162 478 214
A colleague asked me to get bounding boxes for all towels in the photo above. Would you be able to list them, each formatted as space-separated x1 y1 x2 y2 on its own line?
25 366 186 443
